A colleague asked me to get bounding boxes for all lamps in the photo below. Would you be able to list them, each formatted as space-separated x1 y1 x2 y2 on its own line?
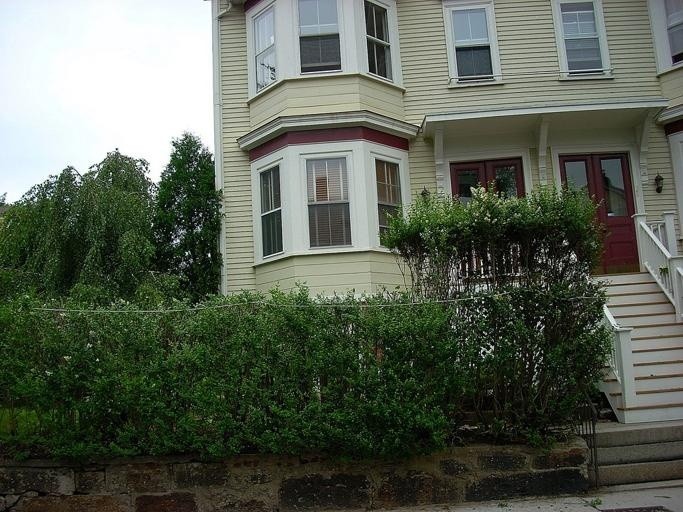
654 170 665 192
